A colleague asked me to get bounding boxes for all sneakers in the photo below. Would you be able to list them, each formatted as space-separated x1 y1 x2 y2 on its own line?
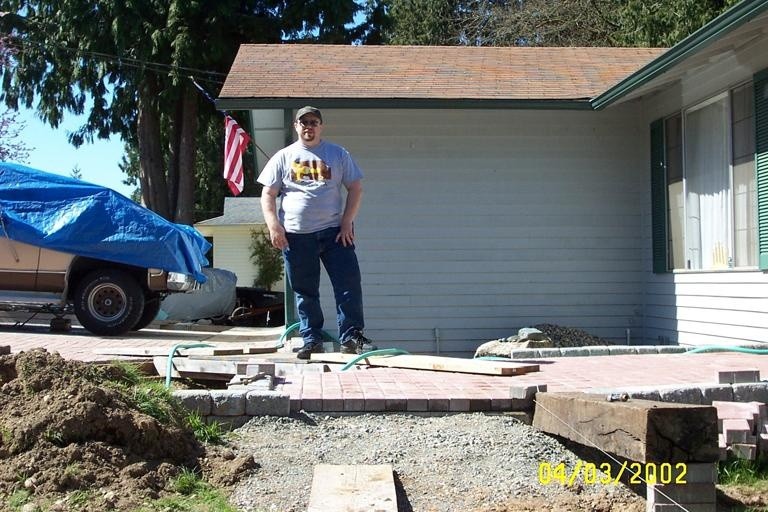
340 333 378 354
296 343 324 359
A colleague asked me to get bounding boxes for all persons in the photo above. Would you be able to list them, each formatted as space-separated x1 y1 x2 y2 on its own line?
255 104 379 361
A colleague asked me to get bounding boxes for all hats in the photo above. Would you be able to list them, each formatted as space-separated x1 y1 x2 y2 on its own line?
296 105 323 124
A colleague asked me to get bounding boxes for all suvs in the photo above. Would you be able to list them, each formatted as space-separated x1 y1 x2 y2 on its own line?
0 162 212 336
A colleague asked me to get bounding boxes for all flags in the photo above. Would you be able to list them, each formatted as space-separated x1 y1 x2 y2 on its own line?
199 88 252 198
